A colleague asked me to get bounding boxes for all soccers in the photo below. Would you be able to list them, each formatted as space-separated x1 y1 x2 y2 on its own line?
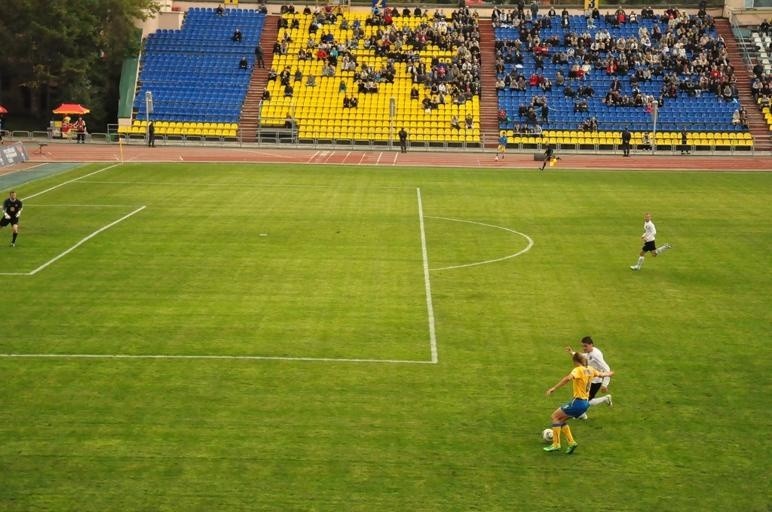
542 429 553 442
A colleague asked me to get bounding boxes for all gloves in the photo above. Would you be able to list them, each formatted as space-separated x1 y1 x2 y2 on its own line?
5 215 11 219
16 212 20 218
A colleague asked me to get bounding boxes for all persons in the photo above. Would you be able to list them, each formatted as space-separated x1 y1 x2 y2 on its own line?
628 212 671 271
73 115 86 144
679 126 690 156
63 116 72 143
1 191 24 247
621 127 632 156
398 127 407 154
540 350 615 456
537 140 561 172
563 335 612 423
148 122 156 148
640 132 652 152
496 132 506 160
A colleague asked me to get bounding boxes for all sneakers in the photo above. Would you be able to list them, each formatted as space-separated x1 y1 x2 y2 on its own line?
630 265 640 271
664 243 672 249
606 394 613 407
543 444 561 452
565 441 578 454
573 412 588 420
10 242 15 247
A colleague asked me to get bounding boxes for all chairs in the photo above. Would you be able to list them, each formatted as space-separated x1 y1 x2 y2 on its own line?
117 5 481 149
495 10 771 148
